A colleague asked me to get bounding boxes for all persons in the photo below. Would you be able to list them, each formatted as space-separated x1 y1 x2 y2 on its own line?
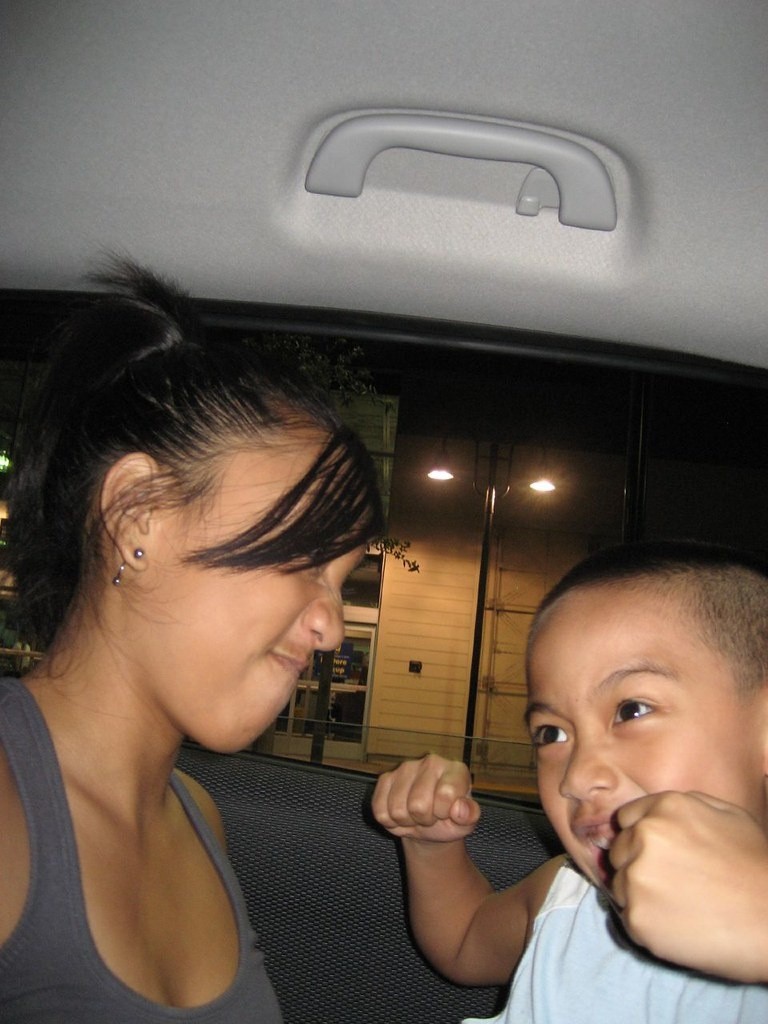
371 539 768 1024
0 243 384 1024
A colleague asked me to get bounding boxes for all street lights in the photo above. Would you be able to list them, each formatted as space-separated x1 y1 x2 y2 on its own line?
426 425 554 768
